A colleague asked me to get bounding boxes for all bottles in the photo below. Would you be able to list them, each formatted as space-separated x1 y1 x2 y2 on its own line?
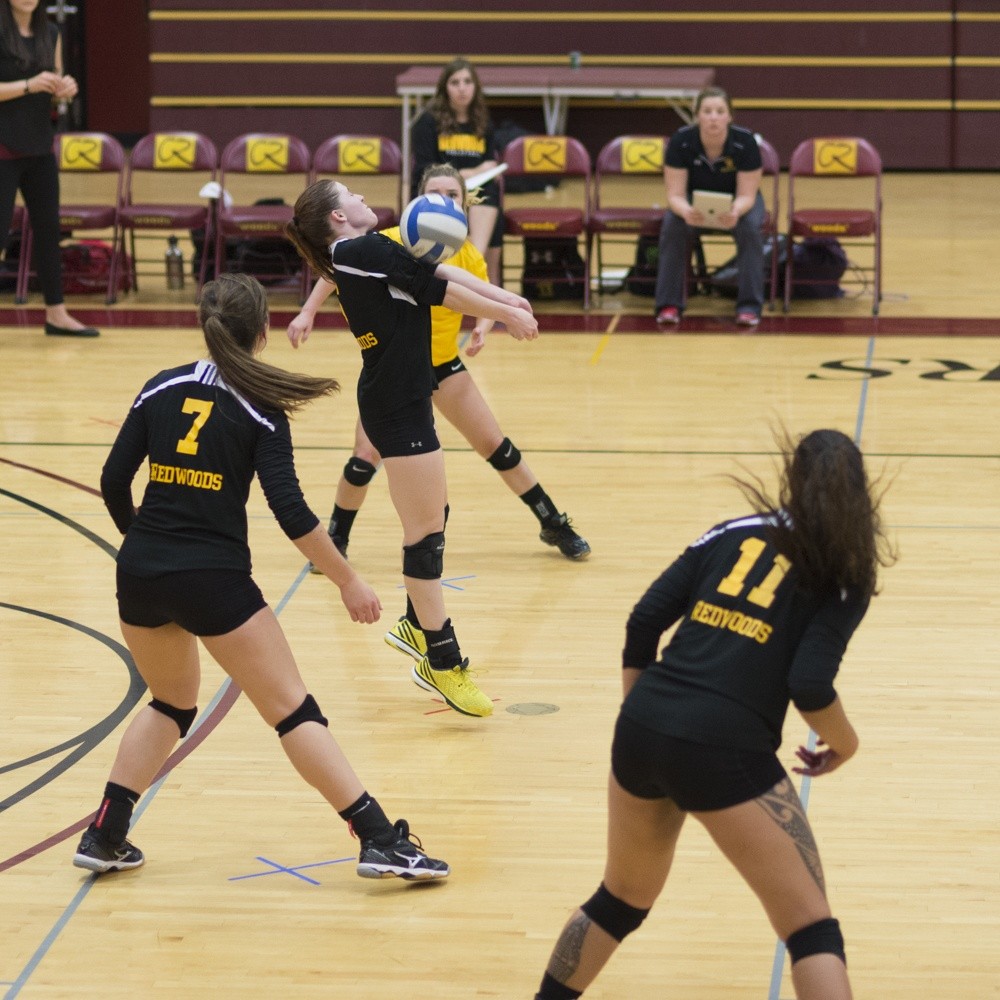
164 236 184 290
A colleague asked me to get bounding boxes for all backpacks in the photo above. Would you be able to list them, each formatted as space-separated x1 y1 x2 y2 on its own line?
519 230 588 304
600 227 713 299
3 227 43 293
702 232 802 302
778 236 849 299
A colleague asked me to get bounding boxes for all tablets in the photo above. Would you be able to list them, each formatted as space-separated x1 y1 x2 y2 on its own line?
693 190 733 228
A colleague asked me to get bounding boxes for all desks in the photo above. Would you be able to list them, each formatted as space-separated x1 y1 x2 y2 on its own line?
394 62 719 220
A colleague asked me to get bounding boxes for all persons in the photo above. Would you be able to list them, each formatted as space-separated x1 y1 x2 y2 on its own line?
0 1 100 338
654 85 767 326
284 165 591 575
407 58 501 254
282 179 540 718
70 274 448 882
534 428 879 1000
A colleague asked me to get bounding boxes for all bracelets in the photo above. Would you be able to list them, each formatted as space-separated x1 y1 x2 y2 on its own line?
24 78 29 95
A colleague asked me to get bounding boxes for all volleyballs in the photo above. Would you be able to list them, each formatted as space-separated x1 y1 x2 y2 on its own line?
400 193 468 262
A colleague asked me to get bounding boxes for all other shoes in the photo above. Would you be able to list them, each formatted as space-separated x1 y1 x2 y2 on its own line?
45 320 101 337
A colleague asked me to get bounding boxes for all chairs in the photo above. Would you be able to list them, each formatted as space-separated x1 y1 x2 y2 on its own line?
0 131 885 314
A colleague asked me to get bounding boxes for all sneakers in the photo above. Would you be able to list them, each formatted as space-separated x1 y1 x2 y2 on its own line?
410 652 494 719
656 305 680 324
539 512 592 561
357 819 450 881
384 616 428 662
309 533 350 574
72 822 144 872
736 313 759 327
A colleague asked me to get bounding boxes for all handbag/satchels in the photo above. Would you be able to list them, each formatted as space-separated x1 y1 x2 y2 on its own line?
59 237 134 293
195 197 303 287
